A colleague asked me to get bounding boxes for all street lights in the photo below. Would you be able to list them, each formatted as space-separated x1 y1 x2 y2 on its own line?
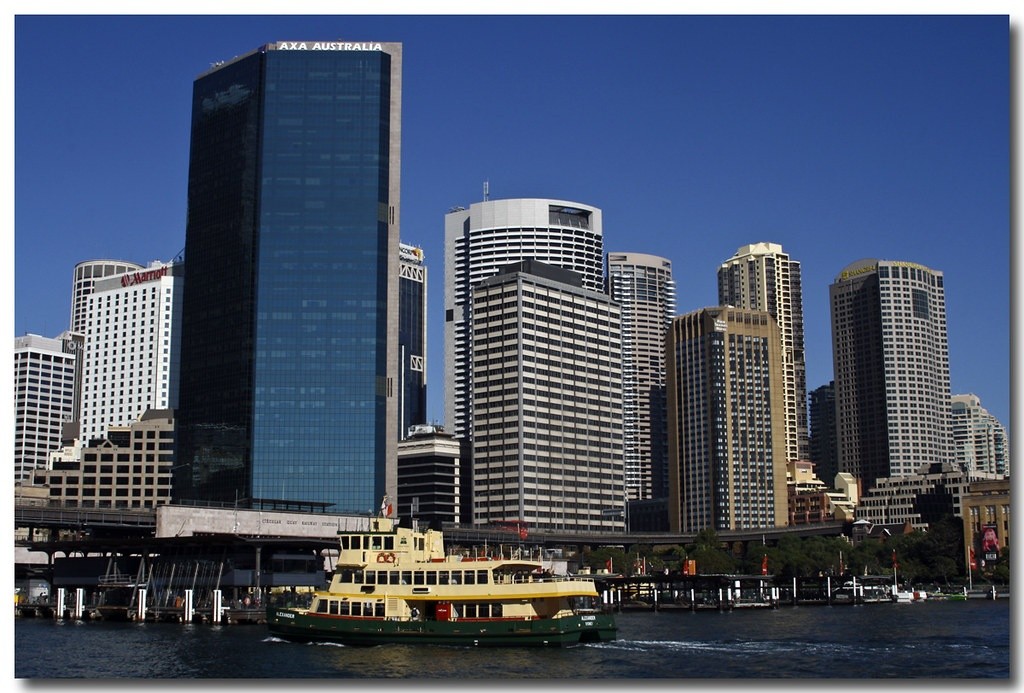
168 462 190 504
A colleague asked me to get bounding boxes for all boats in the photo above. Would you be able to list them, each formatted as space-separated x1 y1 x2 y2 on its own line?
830 574 894 605
259 496 619 651
